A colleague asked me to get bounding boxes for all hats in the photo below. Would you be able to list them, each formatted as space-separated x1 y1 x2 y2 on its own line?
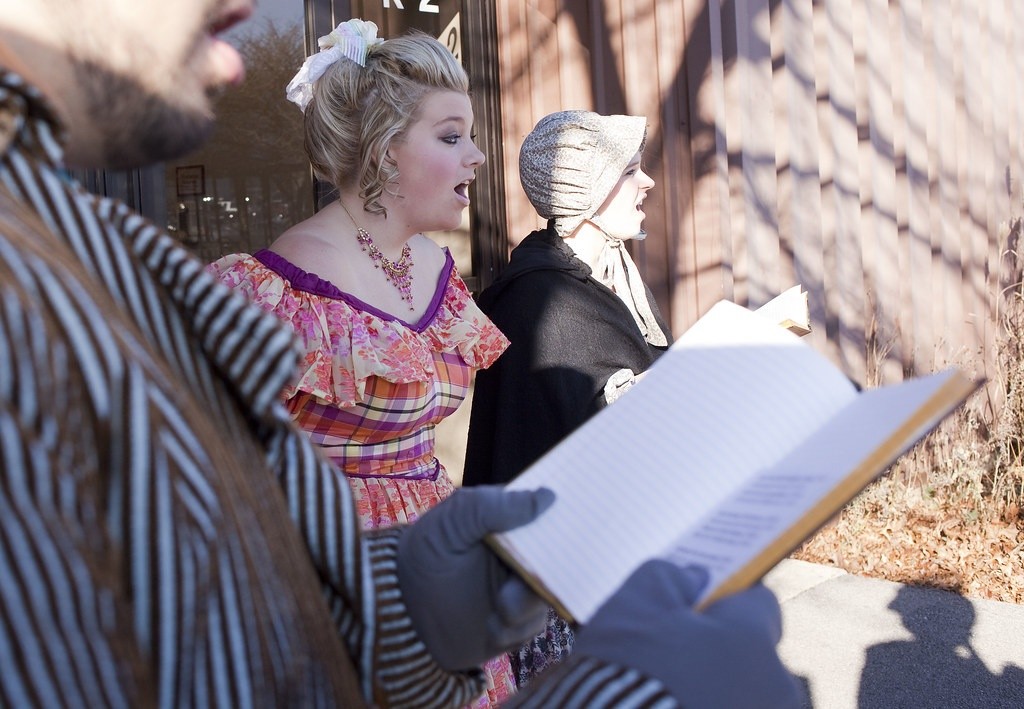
518 110 648 238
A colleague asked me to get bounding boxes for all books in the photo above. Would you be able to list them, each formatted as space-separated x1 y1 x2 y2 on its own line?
755 284 813 339
484 299 993 644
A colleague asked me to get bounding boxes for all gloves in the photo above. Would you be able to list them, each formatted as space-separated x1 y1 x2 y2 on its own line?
395 485 553 674
523 559 801 709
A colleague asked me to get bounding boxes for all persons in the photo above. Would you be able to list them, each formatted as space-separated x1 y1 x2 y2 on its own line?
339 199 420 311
1 0 749 708
461 110 675 686
198 17 520 707
